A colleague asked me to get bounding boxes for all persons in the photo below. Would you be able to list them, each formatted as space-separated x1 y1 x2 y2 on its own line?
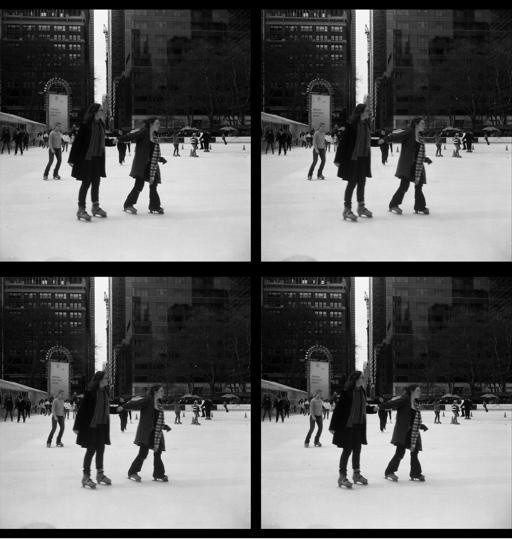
377 382 429 482
381 115 435 217
328 370 377 490
67 102 116 223
123 384 171 484
331 103 381 221
264 120 494 183
116 117 168 216
1 120 232 180
262 388 489 448
0 389 230 449
71 371 122 489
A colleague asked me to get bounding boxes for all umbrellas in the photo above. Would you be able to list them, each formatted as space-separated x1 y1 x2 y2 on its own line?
219 393 240 401
481 126 500 133
481 392 497 401
219 125 238 133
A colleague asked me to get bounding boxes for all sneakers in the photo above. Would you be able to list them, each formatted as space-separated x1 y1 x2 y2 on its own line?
389 203 402 213
410 473 425 479
153 474 169 480
414 205 429 213
307 174 325 179
304 441 321 446
385 470 398 480
43 174 60 180
148 206 164 212
128 471 142 480
47 441 64 446
124 203 137 213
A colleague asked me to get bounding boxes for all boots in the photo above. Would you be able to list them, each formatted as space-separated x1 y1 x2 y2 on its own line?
81 472 96 487
353 472 368 484
343 206 358 220
91 207 106 216
337 477 353 487
356 206 373 216
77 211 92 220
96 472 112 484
